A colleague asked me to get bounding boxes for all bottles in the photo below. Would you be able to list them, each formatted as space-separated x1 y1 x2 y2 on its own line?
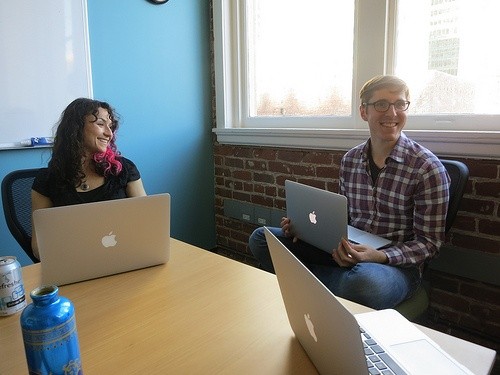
20 284 83 375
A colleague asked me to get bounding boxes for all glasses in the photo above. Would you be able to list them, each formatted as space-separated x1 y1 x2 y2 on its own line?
366 98 411 113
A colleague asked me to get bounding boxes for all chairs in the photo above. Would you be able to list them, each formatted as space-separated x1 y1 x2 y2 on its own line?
393 159 469 322
2 170 42 263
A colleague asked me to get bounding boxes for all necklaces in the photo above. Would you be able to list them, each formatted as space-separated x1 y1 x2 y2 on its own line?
77 166 96 191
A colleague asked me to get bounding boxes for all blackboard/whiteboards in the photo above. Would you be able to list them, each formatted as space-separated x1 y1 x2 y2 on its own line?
0 0 94 150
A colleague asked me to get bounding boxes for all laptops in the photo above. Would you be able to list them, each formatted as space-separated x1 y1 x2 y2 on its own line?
32 192 171 286
263 225 475 375
284 179 393 256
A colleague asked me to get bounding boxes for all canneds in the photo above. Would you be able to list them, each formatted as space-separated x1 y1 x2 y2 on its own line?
0 256 27 317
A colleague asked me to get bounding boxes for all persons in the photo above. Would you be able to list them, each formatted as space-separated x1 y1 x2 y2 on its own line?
31 97 148 263
249 75 450 310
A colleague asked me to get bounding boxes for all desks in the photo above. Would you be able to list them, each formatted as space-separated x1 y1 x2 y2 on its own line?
0 237 497 375
